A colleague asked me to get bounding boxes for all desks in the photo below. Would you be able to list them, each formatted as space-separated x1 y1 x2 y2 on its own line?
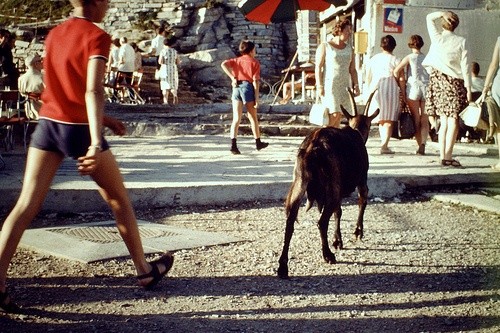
281 66 315 102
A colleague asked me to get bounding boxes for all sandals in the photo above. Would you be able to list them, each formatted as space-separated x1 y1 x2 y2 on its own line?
440 157 462 166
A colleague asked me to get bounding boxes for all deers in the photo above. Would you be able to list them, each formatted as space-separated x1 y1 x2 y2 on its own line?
277 86 381 278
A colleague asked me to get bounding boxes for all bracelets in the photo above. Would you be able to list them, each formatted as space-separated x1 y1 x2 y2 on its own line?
355 84 359 87
316 85 324 88
88 145 103 151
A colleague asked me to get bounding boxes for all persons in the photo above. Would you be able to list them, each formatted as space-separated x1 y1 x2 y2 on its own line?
158 38 181 105
0 0 174 313
0 29 19 89
108 37 143 105
315 19 361 129
151 28 168 70
422 11 472 169
221 40 269 155
283 60 315 102
362 35 405 154
18 54 48 120
395 34 429 155
458 36 500 143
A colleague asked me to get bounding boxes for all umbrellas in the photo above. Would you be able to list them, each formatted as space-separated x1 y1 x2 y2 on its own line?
238 0 347 25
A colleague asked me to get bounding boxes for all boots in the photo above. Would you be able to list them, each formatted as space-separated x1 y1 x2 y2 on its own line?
230 138 242 155
255 137 269 151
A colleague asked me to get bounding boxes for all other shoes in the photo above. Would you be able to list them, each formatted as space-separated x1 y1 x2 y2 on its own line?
415 144 426 155
137 255 173 291
380 148 395 154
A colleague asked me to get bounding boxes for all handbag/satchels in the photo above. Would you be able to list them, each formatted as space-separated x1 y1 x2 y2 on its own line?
459 102 490 130
391 104 416 139
309 95 330 127
